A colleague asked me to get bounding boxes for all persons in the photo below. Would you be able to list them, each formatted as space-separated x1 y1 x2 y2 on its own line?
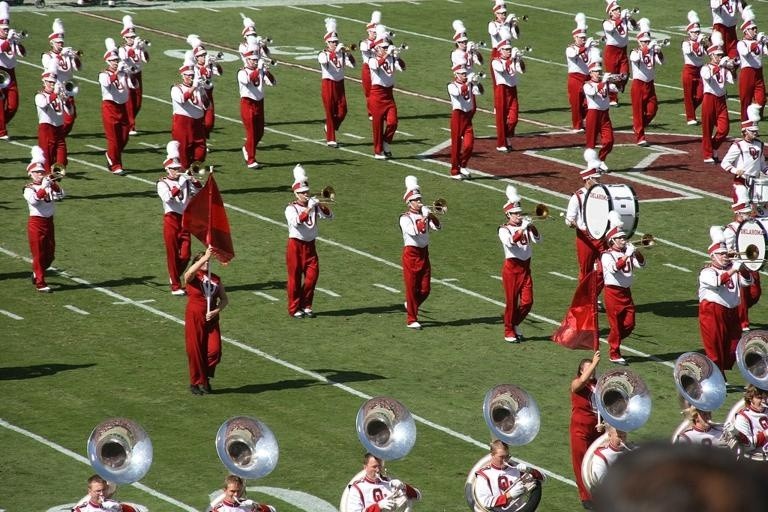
564 167 608 313
182 247 230 397
317 22 405 159
283 180 334 319
72 476 137 511
722 202 762 333
448 0 524 178
348 453 421 512
474 348 768 512
496 199 542 343
22 161 65 292
695 240 756 389
598 225 648 366
719 121 768 218
155 157 204 296
213 475 270 512
565 1 767 175
396 187 443 329
0 26 274 176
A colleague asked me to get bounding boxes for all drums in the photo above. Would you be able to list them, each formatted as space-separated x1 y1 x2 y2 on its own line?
583 183 639 244
745 177 768 208
734 217 767 271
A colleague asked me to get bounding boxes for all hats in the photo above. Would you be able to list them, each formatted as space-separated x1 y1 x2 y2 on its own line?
403 188 424 205
450 0 513 76
502 200 522 215
732 200 752 214
707 241 728 256
292 179 310 193
739 119 759 133
605 225 626 243
321 10 392 50
2 2 262 84
571 1 755 72
579 168 602 179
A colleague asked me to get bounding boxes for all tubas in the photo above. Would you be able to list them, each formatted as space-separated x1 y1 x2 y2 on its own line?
672 330 768 463
464 383 543 512
580 369 654 492
339 398 419 512
75 416 154 507
205 416 280 512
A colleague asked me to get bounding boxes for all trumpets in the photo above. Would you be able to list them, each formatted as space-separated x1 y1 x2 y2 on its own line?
622 233 657 251
465 39 487 50
115 60 138 74
257 35 274 45
4 28 29 43
718 57 739 73
255 57 278 69
597 71 628 81
583 35 607 47
701 35 711 44
464 70 486 81
385 40 409 54
626 7 640 20
191 74 213 89
1 70 11 89
724 244 766 265
50 79 79 95
59 46 84 58
655 39 672 49
130 37 151 48
516 204 550 223
204 51 225 63
753 32 768 46
507 44 532 56
37 162 68 185
302 184 339 206
175 160 213 186
414 196 450 216
510 14 528 23
374 30 395 39
334 43 357 53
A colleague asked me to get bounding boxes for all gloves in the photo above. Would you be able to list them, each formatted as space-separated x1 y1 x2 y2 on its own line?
509 481 527 499
421 206 432 217
42 177 51 190
757 32 768 43
733 261 744 272
53 82 65 95
178 174 192 189
520 216 532 230
378 479 404 511
517 462 534 473
239 499 258 510
102 499 121 510
307 195 320 208
625 244 636 256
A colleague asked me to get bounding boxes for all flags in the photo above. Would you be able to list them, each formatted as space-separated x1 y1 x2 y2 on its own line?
174 172 235 268
548 263 599 352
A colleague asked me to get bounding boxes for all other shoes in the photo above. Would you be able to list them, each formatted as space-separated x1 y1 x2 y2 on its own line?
324 123 391 160
574 100 719 172
0 130 259 174
452 146 511 181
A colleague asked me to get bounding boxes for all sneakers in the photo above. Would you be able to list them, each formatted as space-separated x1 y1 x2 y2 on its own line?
609 356 628 366
170 277 185 295
291 307 316 319
404 300 422 330
504 325 523 343
190 377 213 395
31 269 53 293
